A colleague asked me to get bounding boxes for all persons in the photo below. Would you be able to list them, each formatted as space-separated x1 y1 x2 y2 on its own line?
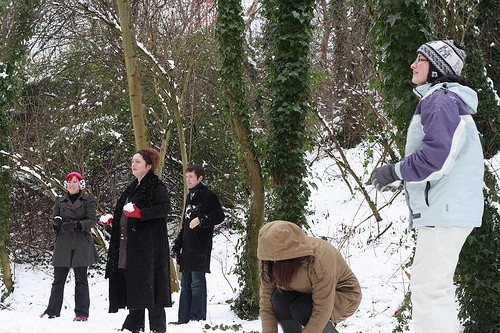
96 148 172 333
170 165 225 325
42 172 96 322
257 221 362 333
371 39 484 333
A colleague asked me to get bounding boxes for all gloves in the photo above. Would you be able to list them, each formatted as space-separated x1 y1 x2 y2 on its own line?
51 218 61 231
368 164 401 191
122 205 141 219
97 213 114 225
61 218 77 230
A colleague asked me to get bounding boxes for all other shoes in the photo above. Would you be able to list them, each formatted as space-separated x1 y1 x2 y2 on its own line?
74 313 87 321
40 313 60 318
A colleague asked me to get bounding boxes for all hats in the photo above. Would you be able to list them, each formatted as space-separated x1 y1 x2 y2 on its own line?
66 172 82 182
416 39 468 77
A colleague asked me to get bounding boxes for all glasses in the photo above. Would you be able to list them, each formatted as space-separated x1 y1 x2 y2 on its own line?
415 57 428 63
